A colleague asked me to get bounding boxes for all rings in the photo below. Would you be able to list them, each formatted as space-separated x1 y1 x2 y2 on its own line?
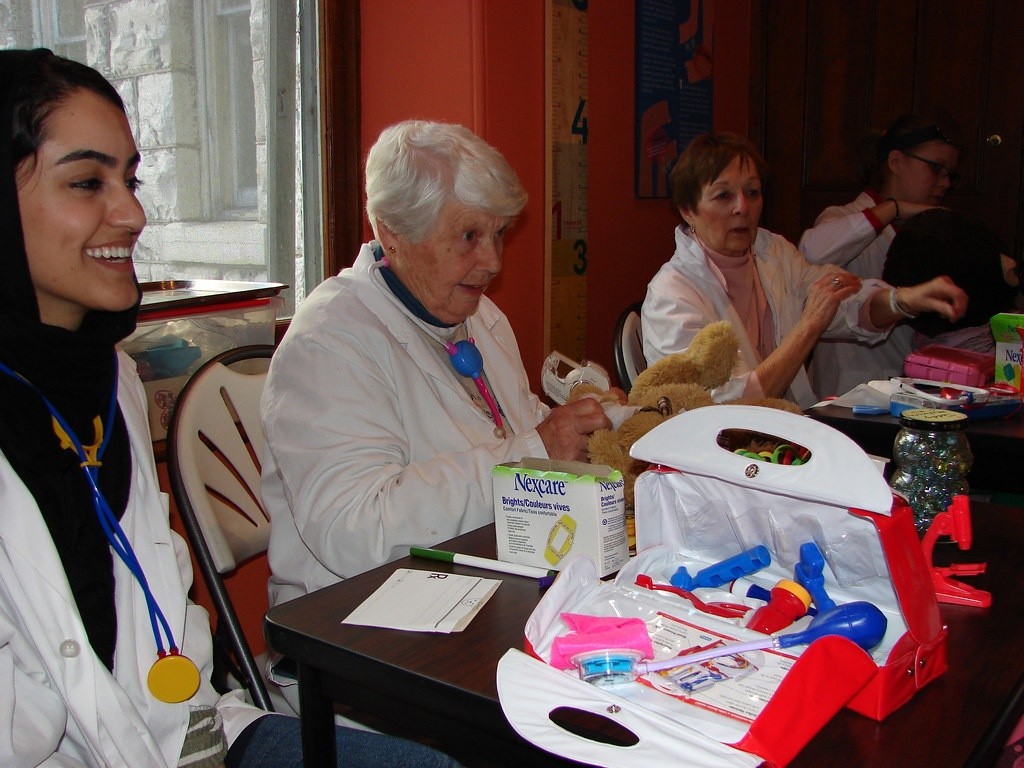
832 278 843 289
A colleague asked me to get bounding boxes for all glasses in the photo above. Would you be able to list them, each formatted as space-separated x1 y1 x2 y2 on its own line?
904 153 959 178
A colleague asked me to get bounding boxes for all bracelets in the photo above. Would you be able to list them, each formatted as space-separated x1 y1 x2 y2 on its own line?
889 287 918 320
883 197 899 219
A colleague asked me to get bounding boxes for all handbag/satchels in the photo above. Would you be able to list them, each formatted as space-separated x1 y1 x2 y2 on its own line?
879 207 1019 339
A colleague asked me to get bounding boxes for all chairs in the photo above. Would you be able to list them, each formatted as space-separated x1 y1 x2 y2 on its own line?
611 300 651 391
159 341 386 733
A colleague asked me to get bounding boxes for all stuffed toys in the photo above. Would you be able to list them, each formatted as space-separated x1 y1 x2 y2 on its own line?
885 208 1021 339
567 320 804 506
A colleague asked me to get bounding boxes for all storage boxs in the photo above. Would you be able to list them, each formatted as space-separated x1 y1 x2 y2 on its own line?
117 294 282 443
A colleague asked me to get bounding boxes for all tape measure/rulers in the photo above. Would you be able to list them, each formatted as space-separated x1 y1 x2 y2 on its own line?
544 0 591 365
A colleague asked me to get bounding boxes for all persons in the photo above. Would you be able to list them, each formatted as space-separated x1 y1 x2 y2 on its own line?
641 132 968 410
797 113 962 402
257 120 614 768
0 48 466 768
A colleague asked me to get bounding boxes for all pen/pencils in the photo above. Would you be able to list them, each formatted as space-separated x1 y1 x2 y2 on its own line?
410 545 554 580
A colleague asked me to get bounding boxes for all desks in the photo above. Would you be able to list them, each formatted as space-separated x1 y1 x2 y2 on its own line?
799 401 1024 496
257 498 1024 768
